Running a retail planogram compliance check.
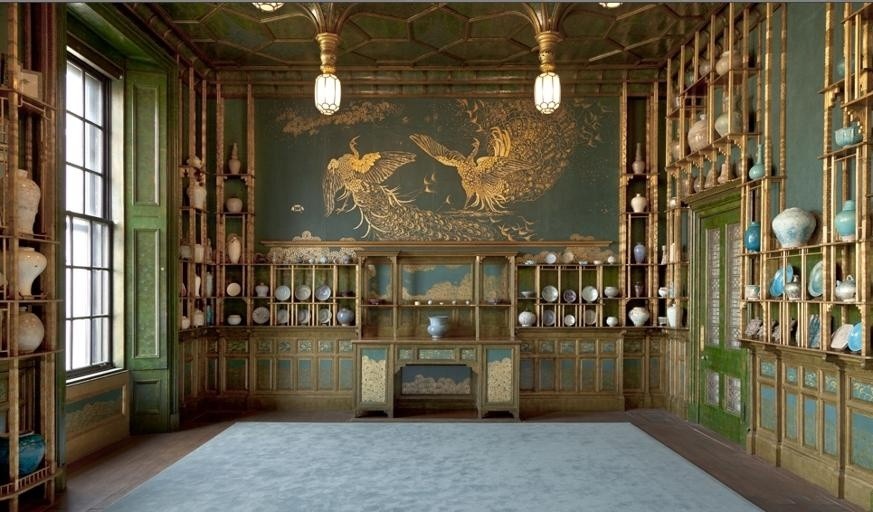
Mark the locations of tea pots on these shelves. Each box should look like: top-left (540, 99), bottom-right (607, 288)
top-left (835, 274), bottom-right (857, 302)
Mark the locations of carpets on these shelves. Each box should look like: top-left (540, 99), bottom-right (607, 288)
top-left (89, 421), bottom-right (763, 511)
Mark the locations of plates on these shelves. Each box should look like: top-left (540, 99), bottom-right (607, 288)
top-left (848, 321), bottom-right (862, 352)
top-left (277, 309), bottom-right (290, 324)
top-left (252, 306), bottom-right (270, 324)
top-left (584, 309), bottom-right (596, 325)
top-left (769, 264), bottom-right (794, 297)
top-left (315, 283), bottom-right (332, 301)
top-left (559, 250), bottom-right (574, 264)
top-left (542, 285), bottom-right (559, 303)
top-left (274, 285), bottom-right (290, 301)
top-left (808, 260), bottom-right (825, 298)
top-left (298, 309), bottom-right (312, 324)
top-left (582, 286), bottom-right (599, 302)
top-left (562, 288), bottom-right (577, 302)
top-left (830, 324), bottom-right (853, 351)
top-left (541, 309), bottom-right (555, 326)
top-left (318, 308), bottom-right (332, 323)
top-left (564, 314), bottom-right (576, 326)
top-left (227, 282), bottom-right (241, 296)
top-left (544, 253), bottom-right (557, 264)
top-left (295, 285), bottom-right (312, 300)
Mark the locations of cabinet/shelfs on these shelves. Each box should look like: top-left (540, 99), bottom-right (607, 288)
top-left (1, 1), bottom-right (63, 511)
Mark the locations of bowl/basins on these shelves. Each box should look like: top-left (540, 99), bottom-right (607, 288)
top-left (341, 290), bottom-right (353, 297)
top-left (521, 291), bottom-right (535, 298)
top-left (834, 124), bottom-right (864, 148)
top-left (368, 298), bottom-right (383, 304)
